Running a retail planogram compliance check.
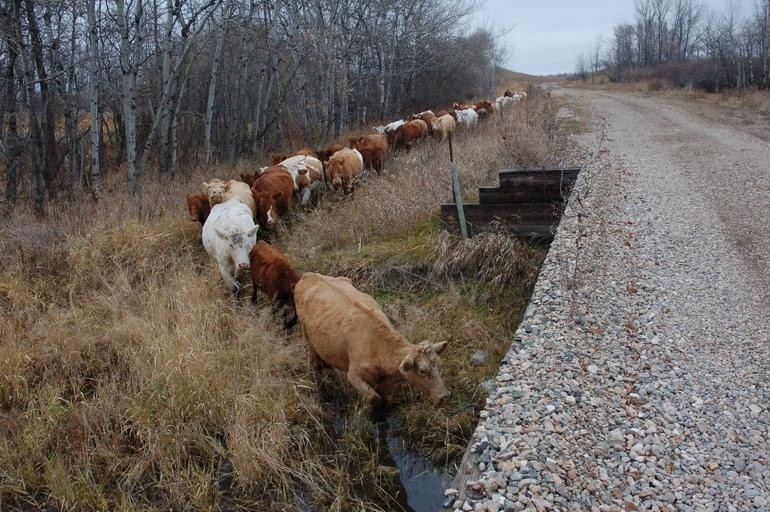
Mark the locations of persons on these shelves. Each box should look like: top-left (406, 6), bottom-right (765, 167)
top-left (504, 89), bottom-right (512, 96)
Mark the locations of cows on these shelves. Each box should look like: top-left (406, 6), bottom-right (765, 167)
top-left (348, 90), bottom-right (525, 179)
top-left (250, 239), bottom-right (303, 330)
top-left (186, 142), bottom-right (363, 225)
top-left (292, 269), bottom-right (451, 419)
top-left (201, 198), bottom-right (259, 303)
